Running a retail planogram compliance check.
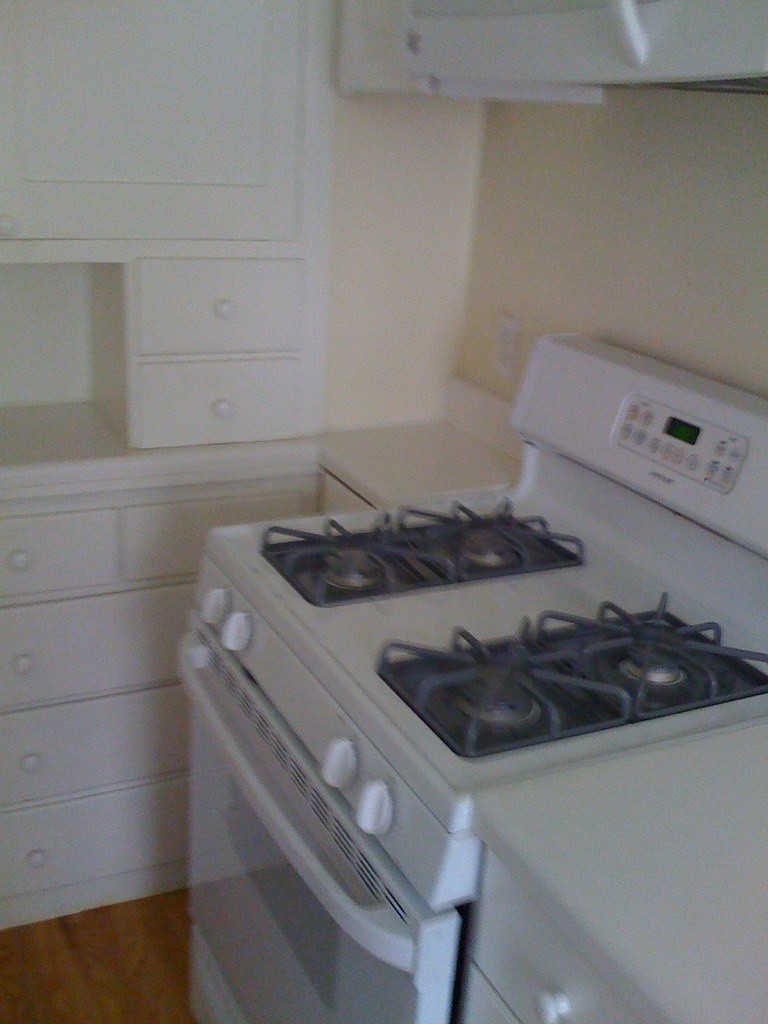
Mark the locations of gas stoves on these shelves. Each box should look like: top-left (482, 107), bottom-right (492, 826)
top-left (252, 328), bottom-right (768, 791)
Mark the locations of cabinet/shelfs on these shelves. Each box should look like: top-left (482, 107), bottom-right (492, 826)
top-left (318, 464), bottom-right (378, 521)
top-left (0, 0), bottom-right (332, 242)
top-left (461, 844), bottom-right (660, 1024)
top-left (133, 254), bottom-right (317, 450)
top-left (0, 473), bottom-right (319, 932)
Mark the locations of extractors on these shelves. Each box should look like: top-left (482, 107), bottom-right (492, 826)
top-left (396, 0), bottom-right (768, 95)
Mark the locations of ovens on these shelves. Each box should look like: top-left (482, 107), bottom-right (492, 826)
top-left (174, 608), bottom-right (467, 1024)
